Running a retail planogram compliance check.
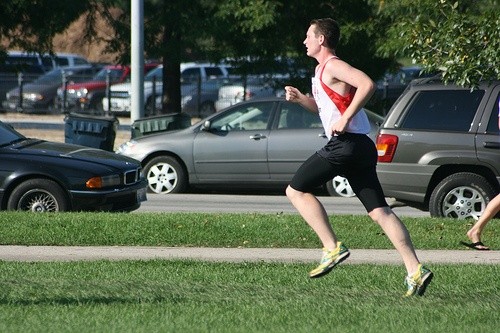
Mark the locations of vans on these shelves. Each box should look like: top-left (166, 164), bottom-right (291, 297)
top-left (0, 51), bottom-right (89, 116)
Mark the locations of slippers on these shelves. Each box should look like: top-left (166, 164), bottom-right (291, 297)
top-left (460, 241), bottom-right (489, 250)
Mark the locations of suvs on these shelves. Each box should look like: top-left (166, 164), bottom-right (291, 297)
top-left (57, 66), bottom-right (131, 113)
top-left (375, 71), bottom-right (500, 218)
top-left (101, 64), bottom-right (229, 116)
top-left (392, 67), bottom-right (443, 85)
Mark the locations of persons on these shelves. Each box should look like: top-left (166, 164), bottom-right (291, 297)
top-left (460, 193), bottom-right (500, 250)
top-left (285, 19), bottom-right (434, 298)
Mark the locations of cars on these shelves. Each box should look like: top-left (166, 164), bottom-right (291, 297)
top-left (2, 66), bottom-right (101, 114)
top-left (144, 77), bottom-right (256, 119)
top-left (116, 98), bottom-right (385, 198)
top-left (0, 121), bottom-right (149, 214)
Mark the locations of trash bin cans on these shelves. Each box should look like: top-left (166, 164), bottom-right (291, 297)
top-left (129, 112), bottom-right (192, 141)
top-left (64, 112), bottom-right (116, 151)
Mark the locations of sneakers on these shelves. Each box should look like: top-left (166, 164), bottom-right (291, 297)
top-left (310, 242), bottom-right (350, 278)
top-left (404, 264), bottom-right (433, 297)
